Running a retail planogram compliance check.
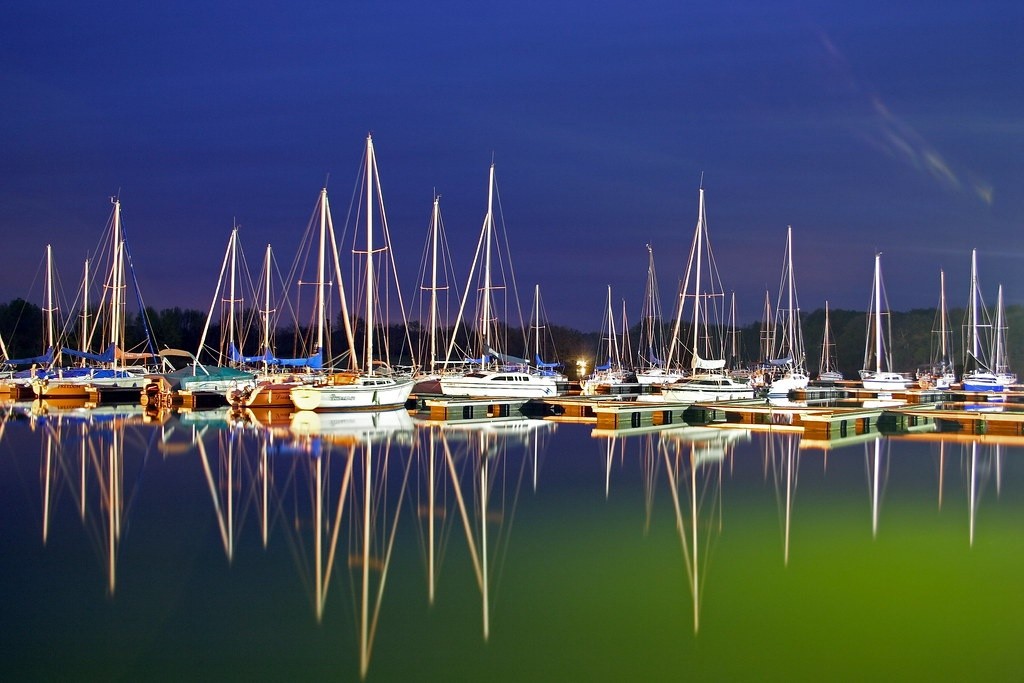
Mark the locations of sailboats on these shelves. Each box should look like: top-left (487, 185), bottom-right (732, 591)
top-left (1, 397), bottom-right (1024, 680)
top-left (1, 131), bottom-right (1024, 409)
